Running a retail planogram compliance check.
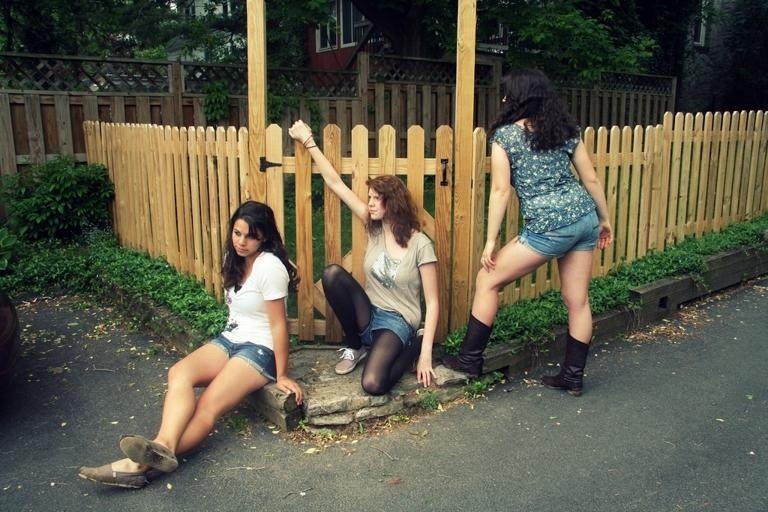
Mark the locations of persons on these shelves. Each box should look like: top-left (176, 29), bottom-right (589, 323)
top-left (77, 201), bottom-right (303, 490)
top-left (287, 119), bottom-right (440, 396)
top-left (440, 65), bottom-right (614, 398)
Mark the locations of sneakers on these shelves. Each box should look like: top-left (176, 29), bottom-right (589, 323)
top-left (416, 328), bottom-right (424, 335)
top-left (78, 434), bottom-right (179, 490)
top-left (335, 346), bottom-right (367, 375)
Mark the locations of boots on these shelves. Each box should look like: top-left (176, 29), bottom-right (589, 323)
top-left (442, 314), bottom-right (492, 377)
top-left (542, 335), bottom-right (589, 396)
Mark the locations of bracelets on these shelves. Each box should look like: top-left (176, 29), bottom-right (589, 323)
top-left (303, 135), bottom-right (317, 149)
